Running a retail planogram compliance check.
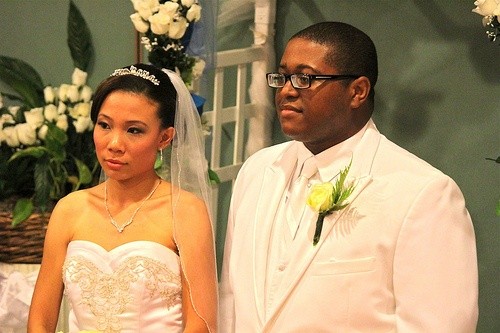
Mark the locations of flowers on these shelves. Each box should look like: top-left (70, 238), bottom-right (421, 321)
top-left (305, 152), bottom-right (358, 245)
top-left (127, 0), bottom-right (206, 91)
top-left (0, 0), bottom-right (109, 227)
top-left (472, 0), bottom-right (499, 41)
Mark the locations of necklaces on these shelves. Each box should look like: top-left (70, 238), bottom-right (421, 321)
top-left (104, 177), bottom-right (161, 233)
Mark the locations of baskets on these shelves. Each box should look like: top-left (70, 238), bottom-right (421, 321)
top-left (0, 201), bottom-right (56, 264)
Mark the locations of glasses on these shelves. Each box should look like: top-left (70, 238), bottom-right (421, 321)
top-left (265, 71), bottom-right (362, 90)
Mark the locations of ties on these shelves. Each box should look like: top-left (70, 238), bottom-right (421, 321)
top-left (286, 154), bottom-right (319, 240)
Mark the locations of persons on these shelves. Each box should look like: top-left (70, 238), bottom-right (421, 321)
top-left (216, 22), bottom-right (479, 333)
top-left (27, 62), bottom-right (223, 333)
